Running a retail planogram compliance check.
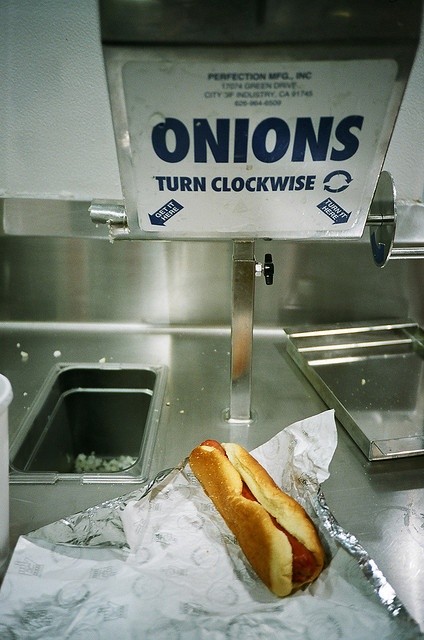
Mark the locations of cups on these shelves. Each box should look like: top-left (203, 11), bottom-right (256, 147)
top-left (1, 373), bottom-right (15, 563)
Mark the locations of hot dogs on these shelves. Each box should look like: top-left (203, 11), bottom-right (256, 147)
top-left (189, 438), bottom-right (328, 601)
